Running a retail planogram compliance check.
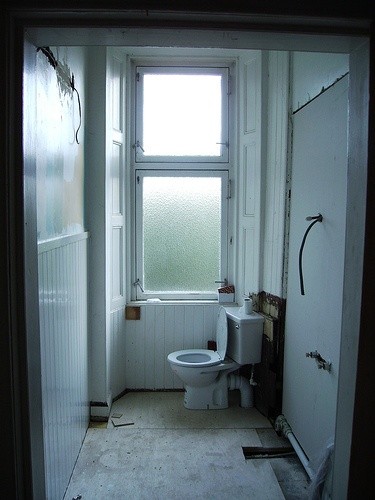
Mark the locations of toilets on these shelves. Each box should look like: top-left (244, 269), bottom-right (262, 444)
top-left (166, 306), bottom-right (265, 409)
